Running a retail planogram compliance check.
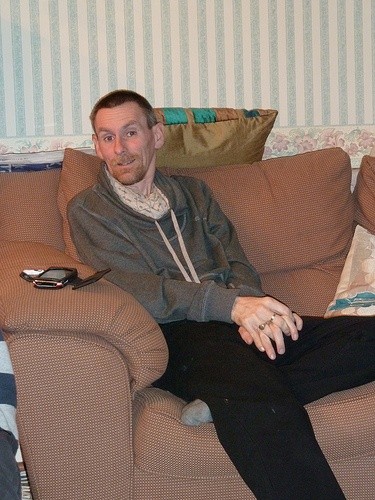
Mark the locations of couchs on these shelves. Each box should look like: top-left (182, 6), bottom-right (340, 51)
top-left (0, 148), bottom-right (375, 500)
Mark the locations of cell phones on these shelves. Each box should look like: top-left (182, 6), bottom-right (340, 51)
top-left (33, 265), bottom-right (78, 290)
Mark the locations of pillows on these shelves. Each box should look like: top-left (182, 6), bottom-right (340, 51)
top-left (152, 107), bottom-right (279, 168)
top-left (323, 224), bottom-right (375, 317)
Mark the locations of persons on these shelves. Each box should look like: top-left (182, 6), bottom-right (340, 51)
top-left (66, 90), bottom-right (375, 500)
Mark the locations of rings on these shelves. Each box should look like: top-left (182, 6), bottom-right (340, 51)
top-left (259, 320), bottom-right (271, 330)
top-left (270, 312), bottom-right (278, 322)
